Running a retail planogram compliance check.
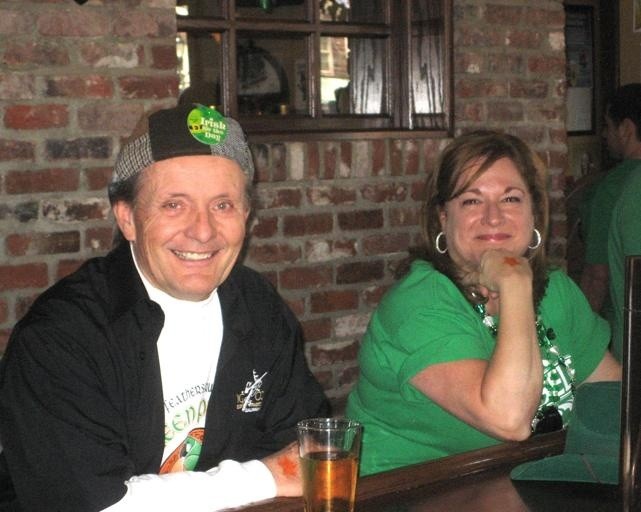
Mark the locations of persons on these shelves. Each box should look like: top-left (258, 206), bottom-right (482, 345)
top-left (333, 130), bottom-right (625, 480)
top-left (575, 82), bottom-right (640, 366)
top-left (605, 164), bottom-right (641, 366)
top-left (0, 99), bottom-right (338, 512)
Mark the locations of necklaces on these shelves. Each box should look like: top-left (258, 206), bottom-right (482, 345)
top-left (468, 289), bottom-right (553, 356)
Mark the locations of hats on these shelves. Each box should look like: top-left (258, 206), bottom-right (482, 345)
top-left (112, 103), bottom-right (255, 185)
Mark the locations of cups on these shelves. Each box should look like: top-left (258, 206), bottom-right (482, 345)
top-left (294, 417), bottom-right (363, 512)
